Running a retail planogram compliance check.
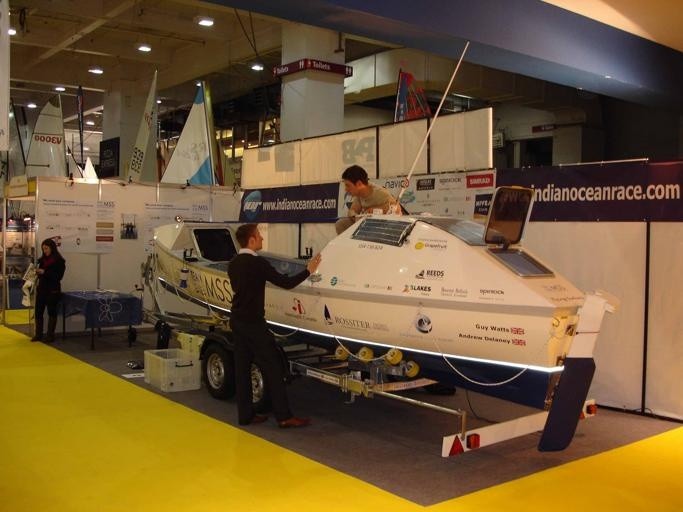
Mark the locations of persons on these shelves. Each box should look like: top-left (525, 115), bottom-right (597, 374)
top-left (333, 164), bottom-right (403, 235)
top-left (225, 221), bottom-right (321, 429)
top-left (29, 238), bottom-right (67, 342)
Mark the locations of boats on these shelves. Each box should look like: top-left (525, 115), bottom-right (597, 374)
top-left (147, 185), bottom-right (621, 457)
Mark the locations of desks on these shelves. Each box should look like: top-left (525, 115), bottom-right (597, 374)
top-left (61, 290), bottom-right (142, 350)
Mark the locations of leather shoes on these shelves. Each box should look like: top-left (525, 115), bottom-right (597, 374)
top-left (244, 412), bottom-right (313, 428)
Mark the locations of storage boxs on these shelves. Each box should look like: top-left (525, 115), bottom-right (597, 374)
top-left (142, 348), bottom-right (202, 394)
